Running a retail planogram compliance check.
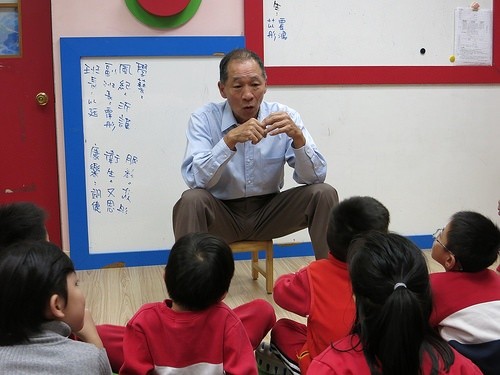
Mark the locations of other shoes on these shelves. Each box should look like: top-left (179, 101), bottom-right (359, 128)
top-left (254, 342), bottom-right (294, 375)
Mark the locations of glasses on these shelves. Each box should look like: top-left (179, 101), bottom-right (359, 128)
top-left (433, 228), bottom-right (455, 257)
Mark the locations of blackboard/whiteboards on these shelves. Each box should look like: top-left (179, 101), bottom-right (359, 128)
top-left (242, 0), bottom-right (500, 85)
top-left (58, 36), bottom-right (500, 272)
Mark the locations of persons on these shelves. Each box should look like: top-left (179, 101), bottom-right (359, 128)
top-left (95, 233), bottom-right (276, 375)
top-left (305, 232), bottom-right (485, 375)
top-left (0, 242), bottom-right (113, 375)
top-left (0, 201), bottom-right (49, 242)
top-left (171, 49), bottom-right (339, 261)
top-left (269, 196), bottom-right (390, 375)
top-left (428, 211), bottom-right (500, 375)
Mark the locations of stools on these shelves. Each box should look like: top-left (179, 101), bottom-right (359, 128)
top-left (229, 240), bottom-right (274, 293)
